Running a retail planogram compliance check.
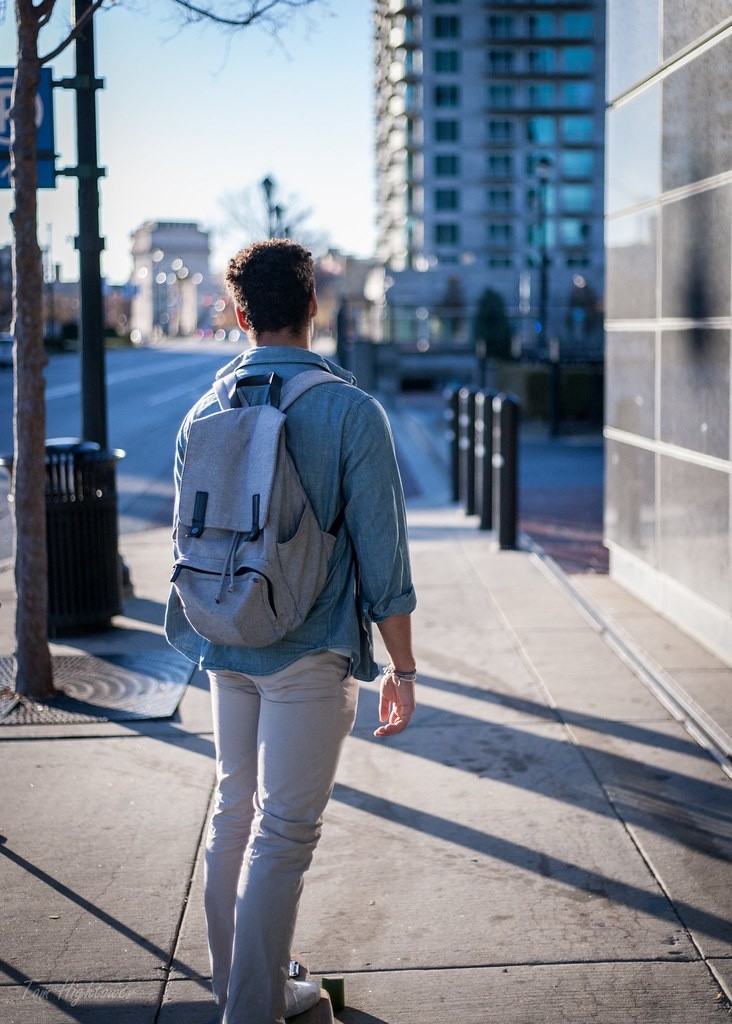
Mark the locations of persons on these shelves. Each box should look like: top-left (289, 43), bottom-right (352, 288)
top-left (156, 240), bottom-right (418, 1024)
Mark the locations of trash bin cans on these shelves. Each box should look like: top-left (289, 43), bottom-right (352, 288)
top-left (0, 438), bottom-right (127, 639)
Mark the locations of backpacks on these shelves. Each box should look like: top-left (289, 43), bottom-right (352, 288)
top-left (165, 371), bottom-right (352, 648)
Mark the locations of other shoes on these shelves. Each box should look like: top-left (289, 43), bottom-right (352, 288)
top-left (285, 981), bottom-right (320, 1018)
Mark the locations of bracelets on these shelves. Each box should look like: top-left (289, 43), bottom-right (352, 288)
top-left (387, 664), bottom-right (417, 685)
